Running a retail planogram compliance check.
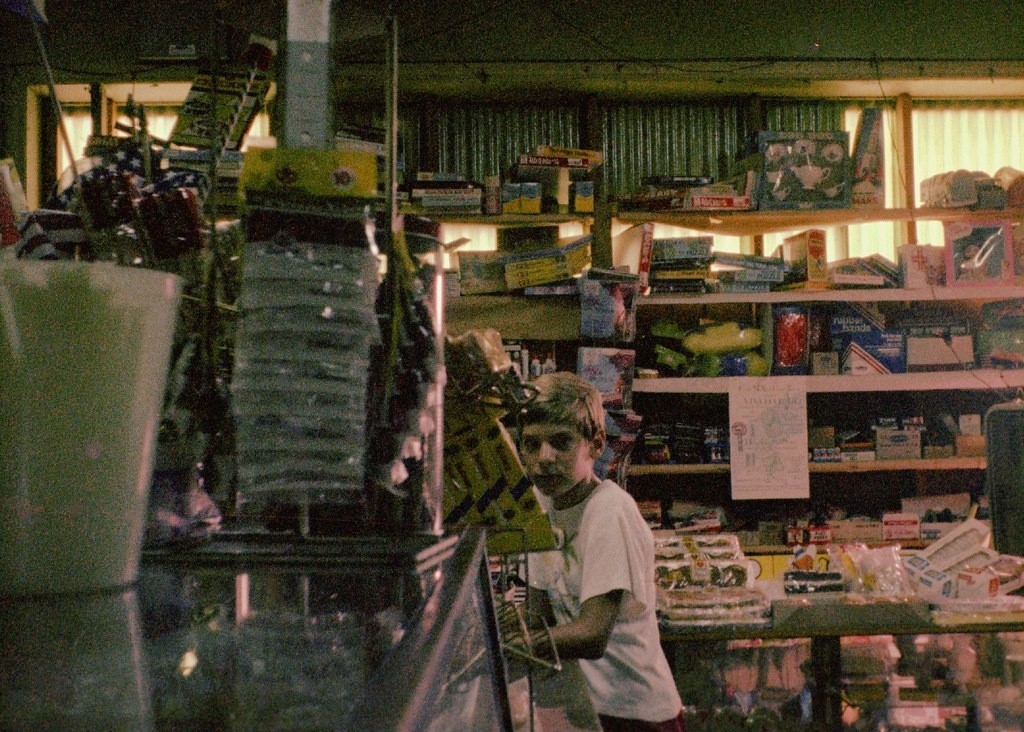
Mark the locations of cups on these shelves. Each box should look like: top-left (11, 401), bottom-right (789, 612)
top-left (0, 589), bottom-right (155, 732)
top-left (0, 260), bottom-right (186, 600)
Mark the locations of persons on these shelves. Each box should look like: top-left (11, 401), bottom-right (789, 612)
top-left (456, 370), bottom-right (686, 732)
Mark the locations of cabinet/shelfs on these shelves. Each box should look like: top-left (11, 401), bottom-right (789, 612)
top-left (431, 206), bottom-right (1024, 477)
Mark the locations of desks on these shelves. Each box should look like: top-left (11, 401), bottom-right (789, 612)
top-left (657, 607), bottom-right (1024, 732)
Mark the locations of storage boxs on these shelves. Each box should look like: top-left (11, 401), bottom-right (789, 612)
top-left (333, 106), bottom-right (1024, 603)
